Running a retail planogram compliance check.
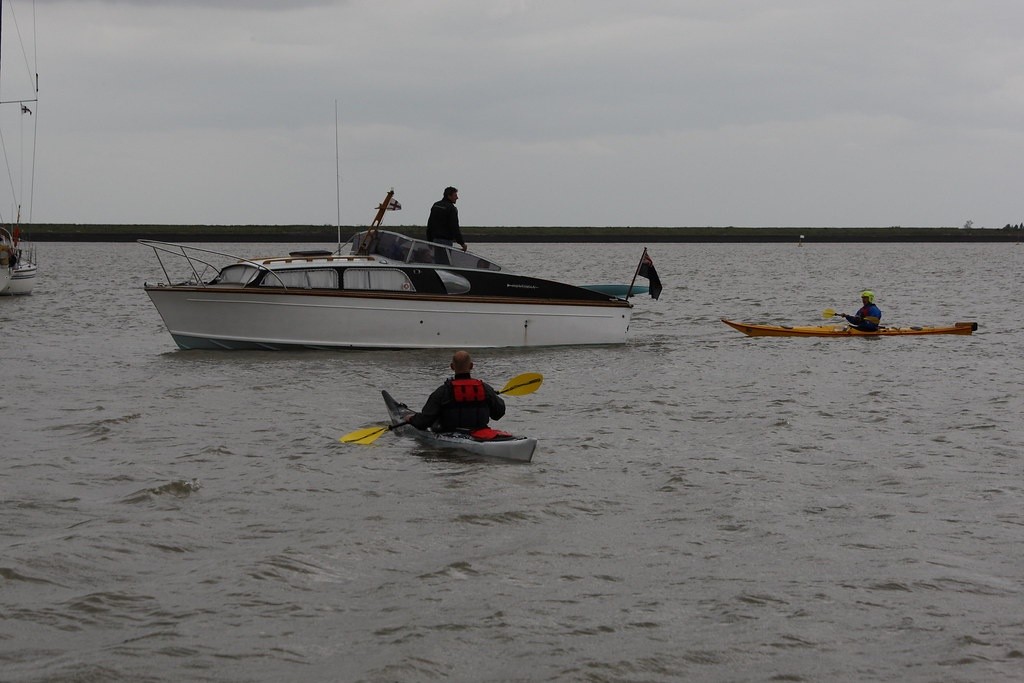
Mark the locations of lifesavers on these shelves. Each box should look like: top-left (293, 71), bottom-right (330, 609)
top-left (0, 246), bottom-right (11, 265)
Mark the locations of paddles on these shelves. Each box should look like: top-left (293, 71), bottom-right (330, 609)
top-left (823, 308), bottom-right (880, 324)
top-left (340, 372), bottom-right (544, 446)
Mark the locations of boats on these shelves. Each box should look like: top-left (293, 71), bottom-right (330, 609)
top-left (718, 314), bottom-right (979, 339)
top-left (381, 389), bottom-right (540, 463)
top-left (132, 98), bottom-right (638, 351)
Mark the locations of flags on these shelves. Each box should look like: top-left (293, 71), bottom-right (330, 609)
top-left (387, 199), bottom-right (401, 211)
top-left (638, 252), bottom-right (663, 301)
top-left (22, 106), bottom-right (32, 115)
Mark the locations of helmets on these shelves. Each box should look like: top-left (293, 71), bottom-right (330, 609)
top-left (861, 290), bottom-right (873, 303)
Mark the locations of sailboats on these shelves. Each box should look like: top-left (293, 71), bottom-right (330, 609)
top-left (0, 2), bottom-right (50, 297)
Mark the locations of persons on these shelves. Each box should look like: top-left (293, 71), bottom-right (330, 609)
top-left (404, 350), bottom-right (506, 435)
top-left (841, 291), bottom-right (882, 331)
top-left (426, 186), bottom-right (467, 265)
